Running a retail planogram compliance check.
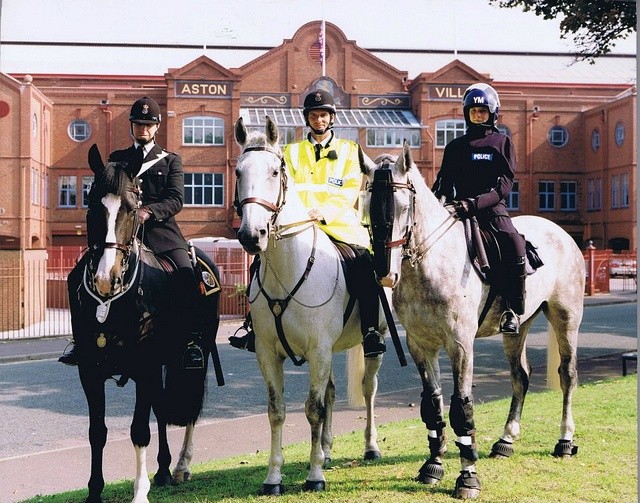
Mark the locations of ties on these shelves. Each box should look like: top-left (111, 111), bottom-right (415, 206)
top-left (315, 144), bottom-right (323, 162)
top-left (130, 145), bottom-right (144, 180)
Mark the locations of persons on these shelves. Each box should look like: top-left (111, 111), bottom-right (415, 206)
top-left (227, 89), bottom-right (386, 358)
top-left (56, 96), bottom-right (206, 369)
top-left (431, 83), bottom-right (527, 336)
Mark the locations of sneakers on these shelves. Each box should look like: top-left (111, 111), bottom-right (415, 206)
top-left (227, 329), bottom-right (256, 353)
top-left (58, 345), bottom-right (78, 363)
top-left (184, 345), bottom-right (208, 367)
top-left (502, 314), bottom-right (520, 332)
top-left (363, 335), bottom-right (386, 358)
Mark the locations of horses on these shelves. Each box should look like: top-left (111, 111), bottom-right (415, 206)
top-left (67, 142), bottom-right (223, 503)
top-left (233, 115), bottom-right (397, 498)
top-left (355, 138), bottom-right (587, 501)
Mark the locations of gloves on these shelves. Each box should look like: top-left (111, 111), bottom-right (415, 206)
top-left (452, 200), bottom-right (474, 215)
top-left (308, 208), bottom-right (324, 223)
top-left (137, 206), bottom-right (153, 224)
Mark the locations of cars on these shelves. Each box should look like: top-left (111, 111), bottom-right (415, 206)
top-left (610, 258), bottom-right (637, 279)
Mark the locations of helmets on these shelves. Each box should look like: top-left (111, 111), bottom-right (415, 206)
top-left (129, 96), bottom-right (162, 125)
top-left (302, 91), bottom-right (336, 117)
top-left (462, 82), bottom-right (501, 127)
